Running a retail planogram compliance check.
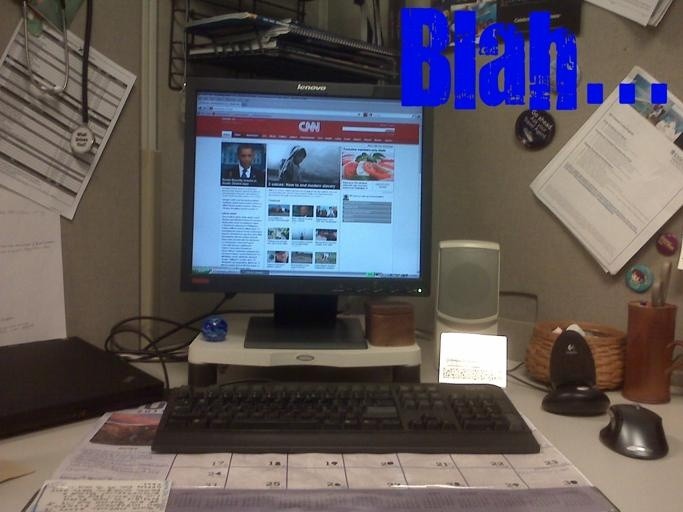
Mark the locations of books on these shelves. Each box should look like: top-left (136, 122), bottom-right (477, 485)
top-left (183, 12), bottom-right (396, 78)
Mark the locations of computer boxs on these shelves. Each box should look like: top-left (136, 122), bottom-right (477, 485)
top-left (188, 311), bottom-right (422, 390)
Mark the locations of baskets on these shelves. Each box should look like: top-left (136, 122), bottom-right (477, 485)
top-left (528, 321), bottom-right (626, 391)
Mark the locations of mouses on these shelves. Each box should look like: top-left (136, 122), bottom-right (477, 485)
top-left (599, 404), bottom-right (669, 460)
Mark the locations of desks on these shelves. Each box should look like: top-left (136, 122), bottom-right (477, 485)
top-left (0, 350), bottom-right (683, 512)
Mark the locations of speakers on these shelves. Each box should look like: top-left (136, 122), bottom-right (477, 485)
top-left (434, 239), bottom-right (501, 368)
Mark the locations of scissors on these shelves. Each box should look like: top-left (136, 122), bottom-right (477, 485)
top-left (651, 261), bottom-right (673, 308)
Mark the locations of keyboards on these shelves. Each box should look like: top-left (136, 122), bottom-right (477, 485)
top-left (151, 383), bottom-right (542, 454)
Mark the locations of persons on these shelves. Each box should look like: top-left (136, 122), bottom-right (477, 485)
top-left (278, 144), bottom-right (308, 184)
top-left (277, 251), bottom-right (287, 264)
top-left (317, 230), bottom-right (327, 239)
top-left (296, 207), bottom-right (311, 215)
top-left (326, 230), bottom-right (337, 241)
top-left (224, 144), bottom-right (264, 187)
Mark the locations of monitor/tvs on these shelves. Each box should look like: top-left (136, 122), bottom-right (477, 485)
top-left (180, 75), bottom-right (434, 351)
